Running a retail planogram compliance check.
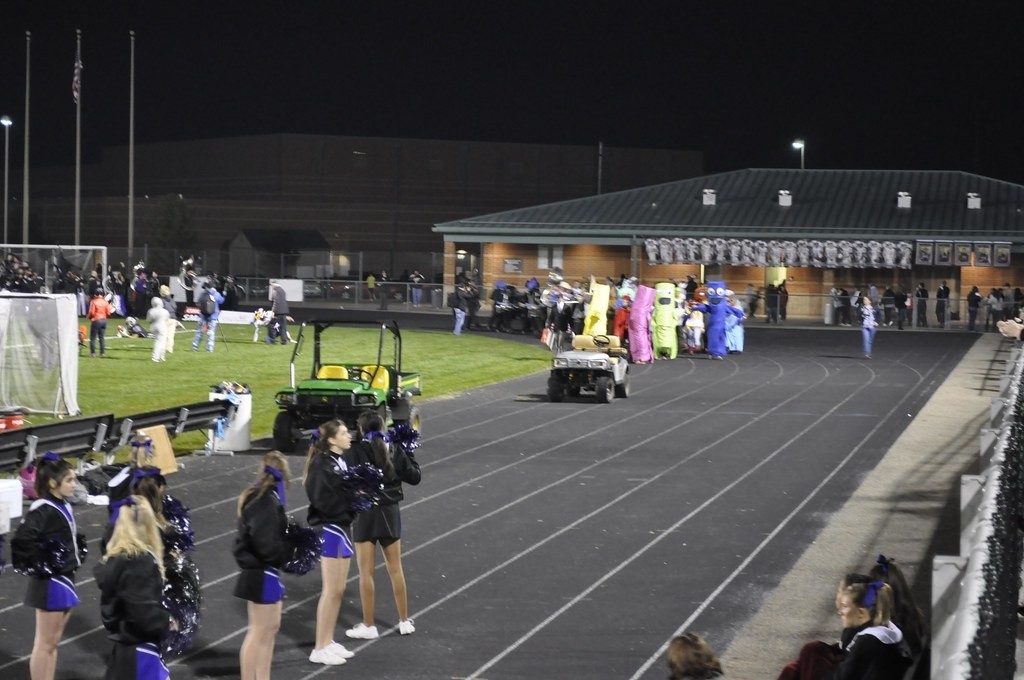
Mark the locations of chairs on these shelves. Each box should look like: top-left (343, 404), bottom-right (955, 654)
top-left (597, 335), bottom-right (621, 364)
top-left (574, 335), bottom-right (595, 350)
top-left (316, 365), bottom-right (349, 379)
top-left (361, 366), bottom-right (389, 393)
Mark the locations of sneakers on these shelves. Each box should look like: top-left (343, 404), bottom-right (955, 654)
top-left (309, 648), bottom-right (347, 666)
top-left (326, 639), bottom-right (355, 658)
top-left (398, 617), bottom-right (416, 635)
top-left (345, 622), bottom-right (379, 639)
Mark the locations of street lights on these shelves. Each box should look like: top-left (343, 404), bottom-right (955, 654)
top-left (0, 115), bottom-right (12, 246)
top-left (791, 138), bottom-right (805, 170)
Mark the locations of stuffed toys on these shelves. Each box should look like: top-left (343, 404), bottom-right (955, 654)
top-left (584, 280), bottom-right (746, 365)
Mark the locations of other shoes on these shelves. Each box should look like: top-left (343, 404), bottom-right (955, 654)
top-left (151, 356), bottom-right (166, 363)
top-left (289, 338), bottom-right (298, 343)
top-left (888, 320), bottom-right (893, 326)
top-left (89, 353), bottom-right (95, 358)
top-left (100, 353), bottom-right (105, 357)
top-left (898, 327), bottom-right (904, 330)
top-left (839, 323), bottom-right (845, 326)
top-left (883, 323), bottom-right (887, 326)
top-left (845, 323), bottom-right (851, 327)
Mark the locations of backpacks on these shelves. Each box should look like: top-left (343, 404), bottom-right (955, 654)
top-left (447, 290), bottom-right (459, 308)
top-left (201, 290), bottom-right (215, 316)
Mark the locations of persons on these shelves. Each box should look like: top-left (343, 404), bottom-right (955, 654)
top-left (0, 254), bottom-right (225, 361)
top-left (748, 280), bottom-right (788, 324)
top-left (94, 434), bottom-right (177, 680)
top-left (921, 248), bottom-right (1008, 263)
top-left (667, 633), bottom-right (723, 680)
top-left (607, 273), bottom-right (627, 286)
top-left (831, 282), bottom-right (928, 330)
top-left (686, 275), bottom-right (697, 299)
top-left (267, 283), bottom-right (297, 344)
top-left (366, 270), bottom-right (389, 310)
top-left (345, 410), bottom-right (422, 639)
top-left (967, 283), bottom-right (1024, 332)
top-left (231, 451), bottom-right (292, 680)
top-left (303, 421), bottom-right (356, 666)
top-left (936, 281), bottom-right (950, 328)
top-left (409, 270), bottom-right (424, 308)
top-left (859, 297), bottom-right (881, 359)
top-left (778, 554), bottom-right (925, 680)
top-left (21, 452), bottom-right (80, 680)
top-left (454, 278), bottom-right (585, 354)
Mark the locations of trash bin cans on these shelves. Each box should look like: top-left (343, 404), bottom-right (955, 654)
top-left (208, 392), bottom-right (252, 451)
top-left (824, 303), bottom-right (835, 325)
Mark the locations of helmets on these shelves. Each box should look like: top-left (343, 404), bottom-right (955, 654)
top-left (126, 317), bottom-right (135, 327)
top-left (160, 284), bottom-right (170, 298)
